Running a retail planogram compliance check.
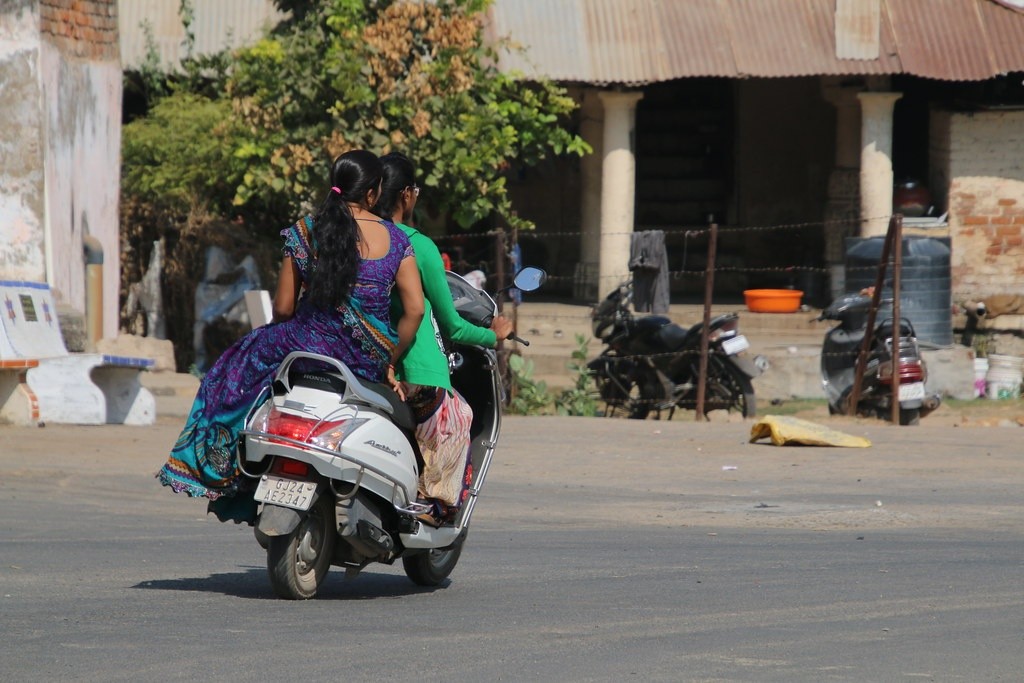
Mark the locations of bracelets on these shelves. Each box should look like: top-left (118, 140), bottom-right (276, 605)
top-left (388, 363), bottom-right (397, 371)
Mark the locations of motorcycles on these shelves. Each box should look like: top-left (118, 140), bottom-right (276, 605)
top-left (587, 281), bottom-right (771, 421)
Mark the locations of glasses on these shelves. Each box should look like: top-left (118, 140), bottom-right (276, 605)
top-left (400, 185), bottom-right (421, 197)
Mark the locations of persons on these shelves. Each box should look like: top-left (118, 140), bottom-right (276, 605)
top-left (155, 150), bottom-right (514, 528)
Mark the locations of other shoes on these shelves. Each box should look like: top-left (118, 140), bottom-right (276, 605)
top-left (208, 491), bottom-right (260, 526)
top-left (425, 496), bottom-right (447, 516)
top-left (415, 496), bottom-right (447, 528)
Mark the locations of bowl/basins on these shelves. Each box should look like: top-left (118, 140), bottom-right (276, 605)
top-left (742, 289), bottom-right (803, 313)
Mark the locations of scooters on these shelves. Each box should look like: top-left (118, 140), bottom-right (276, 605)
top-left (808, 288), bottom-right (942, 427)
top-left (237, 268), bottom-right (549, 600)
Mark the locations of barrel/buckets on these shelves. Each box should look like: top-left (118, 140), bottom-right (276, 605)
top-left (986, 354), bottom-right (1024, 400)
top-left (974, 358), bottom-right (988, 398)
